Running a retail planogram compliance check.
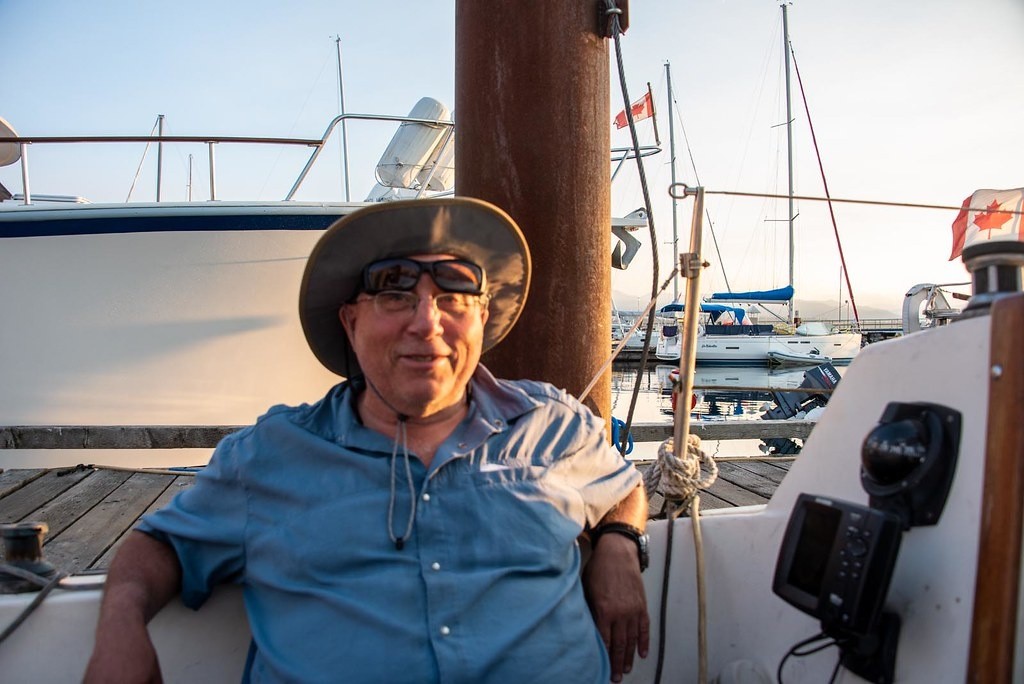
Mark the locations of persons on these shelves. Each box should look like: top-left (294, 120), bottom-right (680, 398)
top-left (79, 196), bottom-right (654, 684)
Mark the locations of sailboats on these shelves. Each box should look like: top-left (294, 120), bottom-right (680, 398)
top-left (654, 0), bottom-right (864, 367)
top-left (654, 362), bottom-right (852, 422)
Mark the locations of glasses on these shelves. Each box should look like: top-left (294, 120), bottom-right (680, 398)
top-left (347, 256), bottom-right (487, 315)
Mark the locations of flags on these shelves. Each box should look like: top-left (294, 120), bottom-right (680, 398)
top-left (947, 188), bottom-right (1024, 262)
top-left (613, 89), bottom-right (656, 129)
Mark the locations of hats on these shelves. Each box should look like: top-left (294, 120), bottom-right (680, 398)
top-left (298, 196), bottom-right (531, 378)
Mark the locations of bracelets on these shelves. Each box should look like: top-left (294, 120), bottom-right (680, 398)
top-left (592, 521), bottom-right (650, 573)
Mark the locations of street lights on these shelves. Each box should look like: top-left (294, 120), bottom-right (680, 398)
top-left (845, 300), bottom-right (850, 332)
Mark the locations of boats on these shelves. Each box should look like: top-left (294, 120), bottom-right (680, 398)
top-left (1, 30), bottom-right (665, 470)
top-left (610, 294), bottom-right (675, 352)
top-left (767, 349), bottom-right (834, 365)
